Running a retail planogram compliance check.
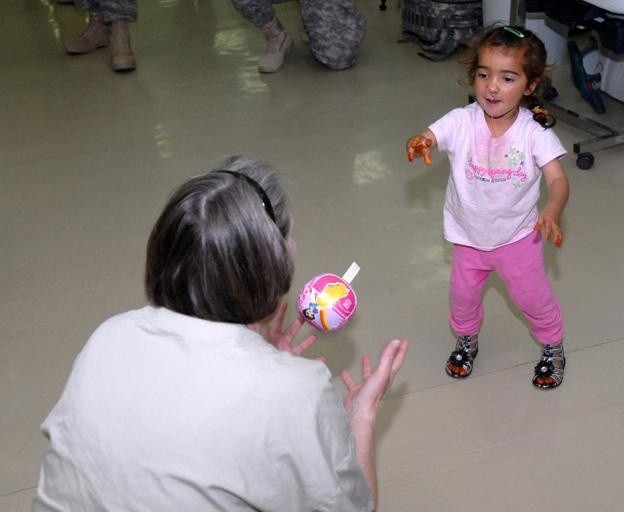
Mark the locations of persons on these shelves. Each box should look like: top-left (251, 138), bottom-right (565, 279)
top-left (65, 0), bottom-right (138, 72)
top-left (230, 0), bottom-right (367, 74)
top-left (30, 153), bottom-right (408, 511)
top-left (406, 23), bottom-right (569, 390)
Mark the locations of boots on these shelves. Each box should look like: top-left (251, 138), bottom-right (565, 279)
top-left (259, 17), bottom-right (295, 74)
top-left (66, 8), bottom-right (110, 54)
top-left (109, 19), bottom-right (136, 74)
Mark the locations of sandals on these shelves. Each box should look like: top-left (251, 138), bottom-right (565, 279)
top-left (446, 336), bottom-right (479, 378)
top-left (532, 343), bottom-right (565, 389)
